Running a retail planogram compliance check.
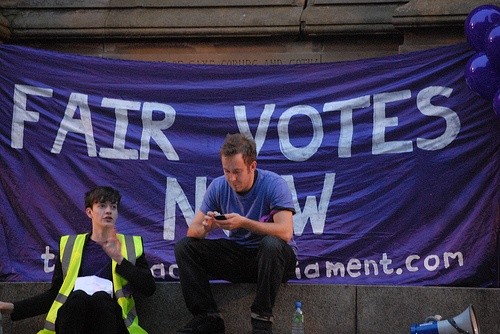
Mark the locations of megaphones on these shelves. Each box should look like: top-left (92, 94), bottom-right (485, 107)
top-left (412, 302), bottom-right (479, 334)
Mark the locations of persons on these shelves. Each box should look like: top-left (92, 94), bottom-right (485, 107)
top-left (0, 186), bottom-right (157, 334)
top-left (174, 134), bottom-right (299, 334)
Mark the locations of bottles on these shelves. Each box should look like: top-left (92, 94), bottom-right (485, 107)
top-left (290, 301), bottom-right (305, 334)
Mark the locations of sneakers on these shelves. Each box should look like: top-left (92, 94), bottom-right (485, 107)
top-left (250, 318), bottom-right (273, 333)
top-left (177, 316), bottom-right (225, 334)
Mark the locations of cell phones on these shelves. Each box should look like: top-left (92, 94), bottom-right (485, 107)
top-left (215, 215), bottom-right (226, 220)
